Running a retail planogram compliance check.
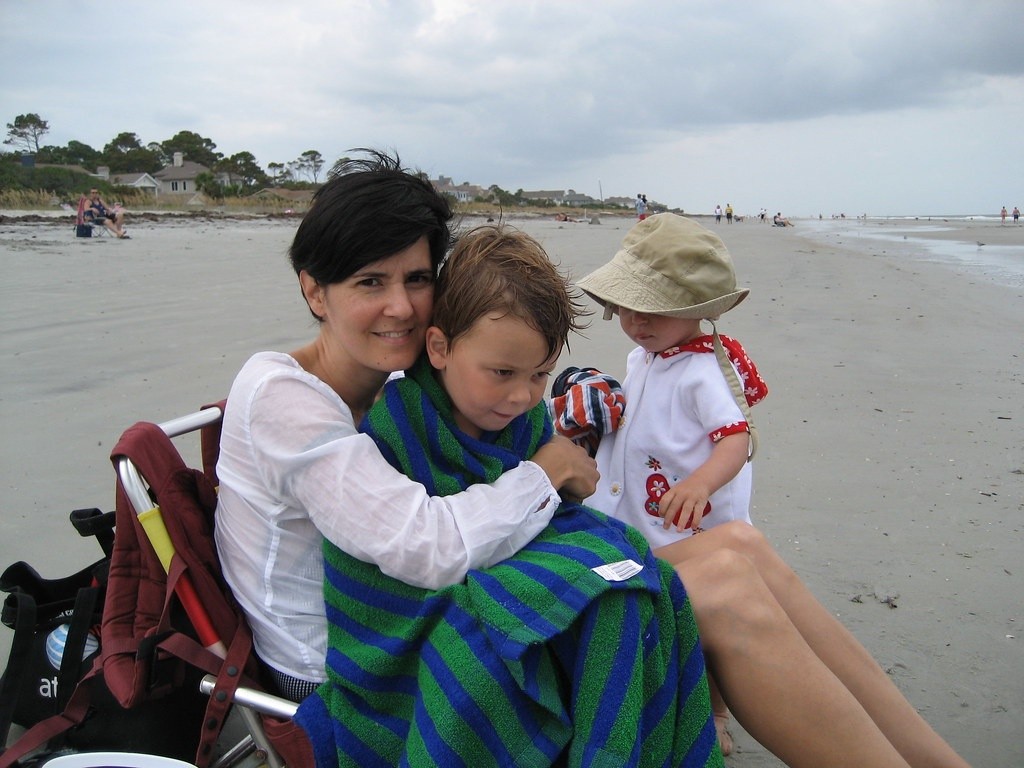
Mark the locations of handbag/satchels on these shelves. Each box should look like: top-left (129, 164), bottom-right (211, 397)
top-left (0, 507), bottom-right (231, 760)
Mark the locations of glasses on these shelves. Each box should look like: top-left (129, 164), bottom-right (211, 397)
top-left (91, 192), bottom-right (98, 194)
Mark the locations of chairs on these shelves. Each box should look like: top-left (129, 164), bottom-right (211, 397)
top-left (115, 399), bottom-right (324, 768)
top-left (79, 196), bottom-right (113, 238)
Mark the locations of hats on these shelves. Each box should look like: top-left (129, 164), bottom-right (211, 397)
top-left (575, 212), bottom-right (751, 321)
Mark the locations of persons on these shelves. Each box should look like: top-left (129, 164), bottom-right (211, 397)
top-left (1011, 207), bottom-right (1020, 221)
top-left (575, 212), bottom-right (768, 753)
top-left (758, 208), bottom-right (767, 224)
top-left (214, 150), bottom-right (970, 768)
top-left (725, 204), bottom-right (733, 224)
top-left (293, 226), bottom-right (721, 768)
top-left (773, 212), bottom-right (794, 227)
top-left (636, 194), bottom-right (648, 220)
top-left (84, 188), bottom-right (129, 239)
top-left (1000, 206), bottom-right (1007, 223)
top-left (715, 205), bottom-right (722, 224)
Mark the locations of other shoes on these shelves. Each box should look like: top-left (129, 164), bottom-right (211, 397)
top-left (120, 231), bottom-right (130, 239)
